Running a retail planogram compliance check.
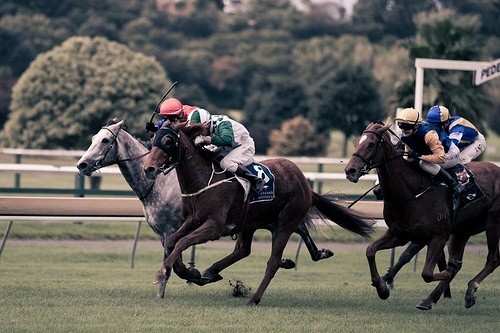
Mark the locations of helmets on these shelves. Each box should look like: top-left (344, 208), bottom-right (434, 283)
top-left (394, 107), bottom-right (423, 125)
top-left (158, 98), bottom-right (184, 115)
top-left (185, 108), bottom-right (212, 127)
top-left (427, 105), bottom-right (451, 123)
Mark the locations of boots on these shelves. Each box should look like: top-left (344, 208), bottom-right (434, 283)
top-left (435, 167), bottom-right (465, 193)
top-left (234, 165), bottom-right (265, 195)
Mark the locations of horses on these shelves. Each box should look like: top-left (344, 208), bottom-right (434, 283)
top-left (345, 122), bottom-right (500, 311)
top-left (75, 117), bottom-right (379, 308)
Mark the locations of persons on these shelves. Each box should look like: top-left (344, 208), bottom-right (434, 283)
top-left (146, 98), bottom-right (265, 197)
top-left (394, 105), bottom-right (487, 211)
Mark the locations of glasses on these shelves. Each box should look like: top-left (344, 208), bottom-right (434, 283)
top-left (398, 122), bottom-right (415, 130)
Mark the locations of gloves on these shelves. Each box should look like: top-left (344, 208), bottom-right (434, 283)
top-left (194, 135), bottom-right (204, 146)
top-left (408, 150), bottom-right (423, 161)
top-left (146, 122), bottom-right (159, 133)
